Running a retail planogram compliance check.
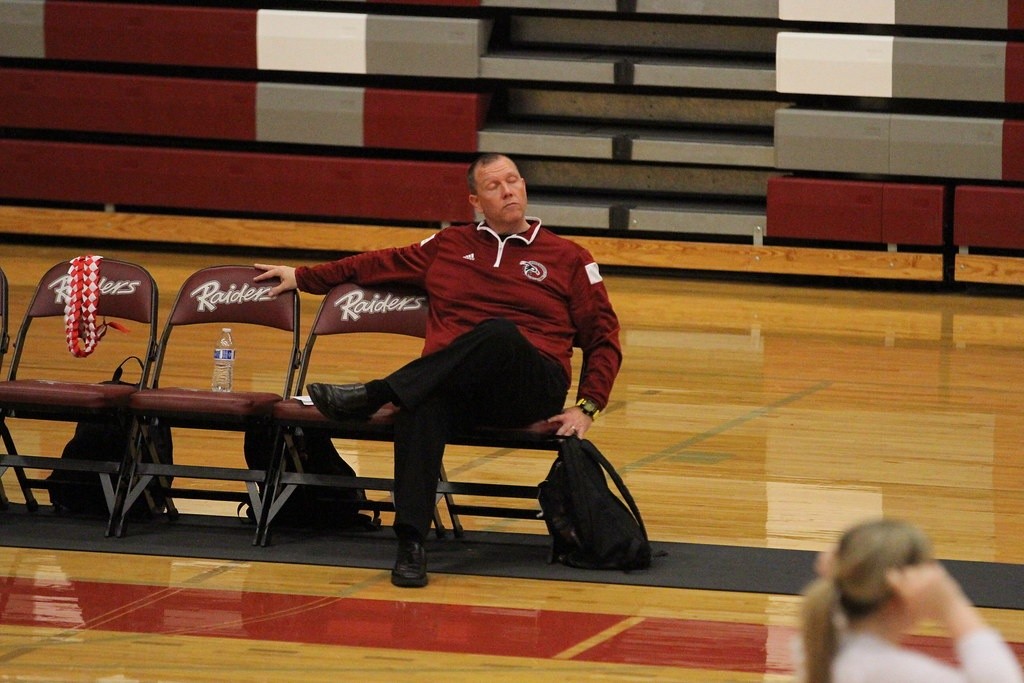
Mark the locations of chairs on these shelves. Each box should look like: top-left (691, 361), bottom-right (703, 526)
top-left (0, 254), bottom-right (589, 565)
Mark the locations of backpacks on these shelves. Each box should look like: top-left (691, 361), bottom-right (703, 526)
top-left (46, 355), bottom-right (173, 519)
top-left (539, 434), bottom-right (652, 571)
top-left (237, 430), bottom-right (368, 538)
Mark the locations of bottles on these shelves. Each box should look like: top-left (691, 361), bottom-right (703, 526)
top-left (211, 327), bottom-right (236, 392)
top-left (752, 224), bottom-right (763, 260)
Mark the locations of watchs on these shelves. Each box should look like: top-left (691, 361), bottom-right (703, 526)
top-left (576, 398), bottom-right (600, 422)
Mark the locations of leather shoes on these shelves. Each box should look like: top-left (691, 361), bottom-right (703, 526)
top-left (306, 382), bottom-right (371, 425)
top-left (391, 540), bottom-right (429, 588)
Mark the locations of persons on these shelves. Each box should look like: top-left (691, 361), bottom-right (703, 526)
top-left (253, 153), bottom-right (625, 589)
top-left (793, 518), bottom-right (1024, 682)
